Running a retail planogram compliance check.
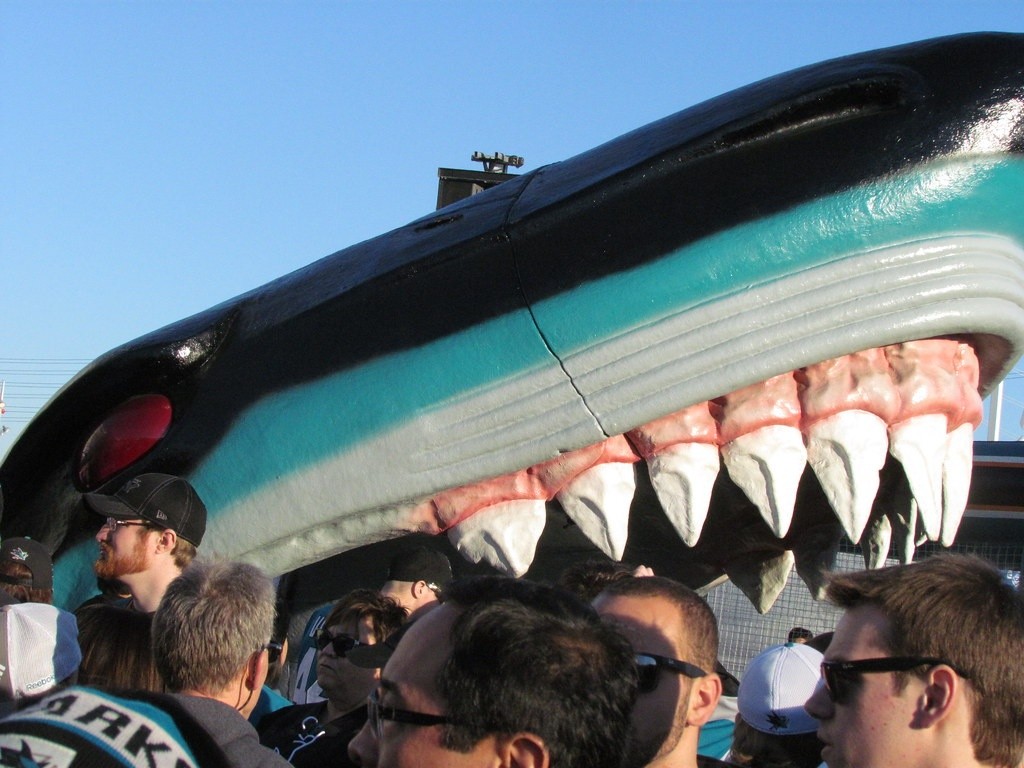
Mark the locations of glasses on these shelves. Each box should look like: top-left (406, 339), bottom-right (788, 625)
top-left (106, 517), bottom-right (160, 531)
top-left (633, 651), bottom-right (708, 692)
top-left (314, 629), bottom-right (373, 658)
top-left (255, 635), bottom-right (283, 663)
top-left (367, 695), bottom-right (513, 740)
top-left (819, 656), bottom-right (972, 705)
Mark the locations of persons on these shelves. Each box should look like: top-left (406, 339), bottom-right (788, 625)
top-left (0, 471), bottom-right (455, 768)
top-left (592, 575), bottom-right (722, 768)
top-left (353, 574), bottom-right (638, 768)
top-left (805, 555), bottom-right (1024, 768)
top-left (733, 626), bottom-right (834, 768)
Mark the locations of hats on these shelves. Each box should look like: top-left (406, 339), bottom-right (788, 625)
top-left (82, 473), bottom-right (207, 548)
top-left (0, 685), bottom-right (227, 768)
top-left (0, 537), bottom-right (53, 590)
top-left (738, 642), bottom-right (827, 735)
top-left (0, 601), bottom-right (82, 699)
top-left (345, 620), bottom-right (419, 668)
top-left (386, 545), bottom-right (452, 603)
